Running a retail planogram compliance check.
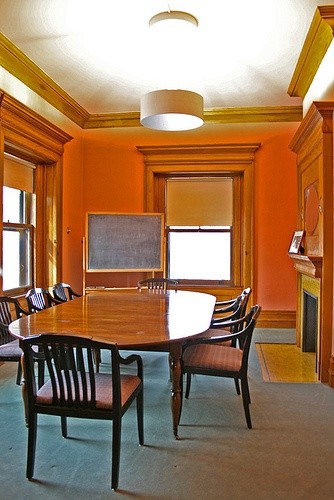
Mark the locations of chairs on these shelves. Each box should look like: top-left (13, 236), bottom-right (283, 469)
top-left (0, 283), bottom-right (92, 386)
top-left (138, 278), bottom-right (178, 289)
top-left (177, 304), bottom-right (261, 429)
top-left (211, 288), bottom-right (252, 350)
top-left (19, 334), bottom-right (144, 491)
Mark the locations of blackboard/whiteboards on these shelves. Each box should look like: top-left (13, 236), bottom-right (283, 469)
top-left (85, 211), bottom-right (165, 273)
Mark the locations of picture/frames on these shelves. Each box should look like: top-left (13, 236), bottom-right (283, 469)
top-left (288, 231), bottom-right (304, 254)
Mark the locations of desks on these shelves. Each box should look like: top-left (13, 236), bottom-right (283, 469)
top-left (8, 289), bottom-right (216, 439)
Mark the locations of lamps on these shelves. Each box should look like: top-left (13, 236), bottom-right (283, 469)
top-left (140, 11), bottom-right (204, 131)
